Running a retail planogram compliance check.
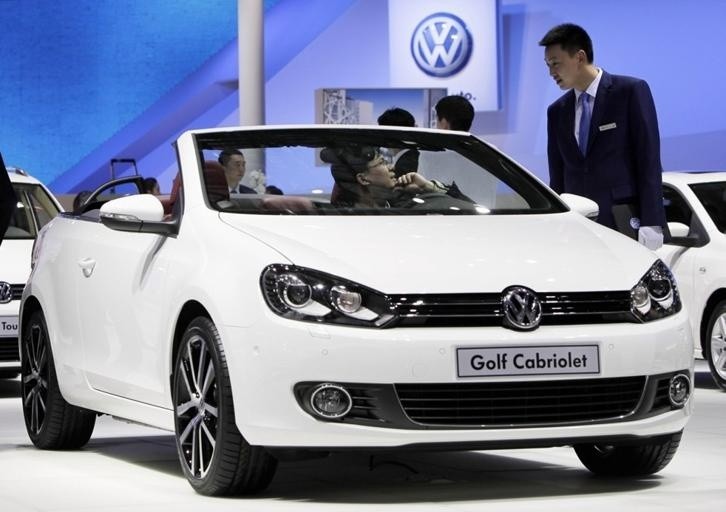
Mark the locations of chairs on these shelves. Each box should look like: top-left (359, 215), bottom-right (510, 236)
top-left (328, 184), bottom-right (357, 210)
top-left (169, 162), bottom-right (231, 210)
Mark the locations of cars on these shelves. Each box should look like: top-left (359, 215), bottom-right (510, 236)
top-left (654, 168), bottom-right (726, 392)
top-left (17, 123), bottom-right (696, 495)
top-left (0, 167), bottom-right (68, 379)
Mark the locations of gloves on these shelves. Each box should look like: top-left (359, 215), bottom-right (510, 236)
top-left (637, 223), bottom-right (665, 252)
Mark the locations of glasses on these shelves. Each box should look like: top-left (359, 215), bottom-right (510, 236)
top-left (361, 156), bottom-right (390, 175)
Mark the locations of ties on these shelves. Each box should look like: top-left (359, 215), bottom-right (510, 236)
top-left (578, 92), bottom-right (594, 159)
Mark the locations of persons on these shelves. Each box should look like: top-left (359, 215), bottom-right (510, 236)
top-left (143, 177), bottom-right (161, 195)
top-left (218, 151), bottom-right (257, 195)
top-left (320, 144), bottom-right (477, 208)
top-left (539, 21), bottom-right (672, 253)
top-left (266, 185), bottom-right (283, 195)
top-left (377, 108), bottom-right (420, 178)
top-left (419, 95), bottom-right (498, 209)
